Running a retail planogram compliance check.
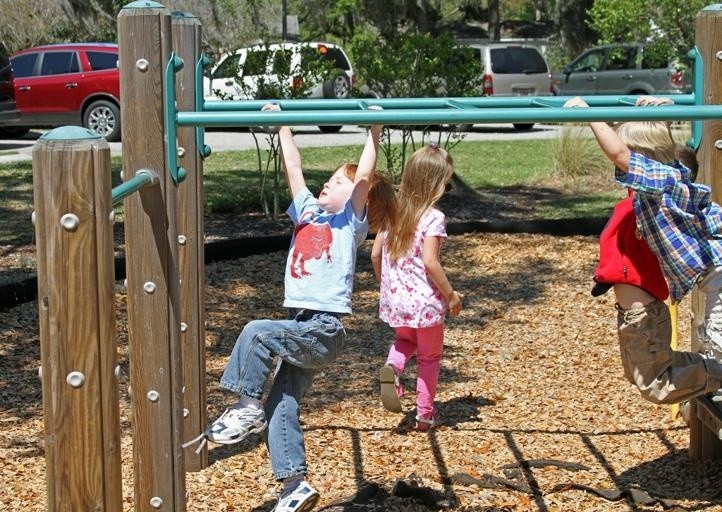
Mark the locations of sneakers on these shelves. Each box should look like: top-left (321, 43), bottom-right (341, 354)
top-left (205, 407), bottom-right (267, 446)
top-left (267, 481), bottom-right (317, 512)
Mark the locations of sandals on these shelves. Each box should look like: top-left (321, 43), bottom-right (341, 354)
top-left (380, 365), bottom-right (401, 415)
top-left (414, 413), bottom-right (443, 431)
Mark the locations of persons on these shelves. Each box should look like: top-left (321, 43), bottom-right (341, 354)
top-left (591, 93), bottom-right (722, 407)
top-left (181, 102), bottom-right (402, 512)
top-left (370, 140), bottom-right (466, 435)
top-left (561, 95), bottom-right (722, 364)
